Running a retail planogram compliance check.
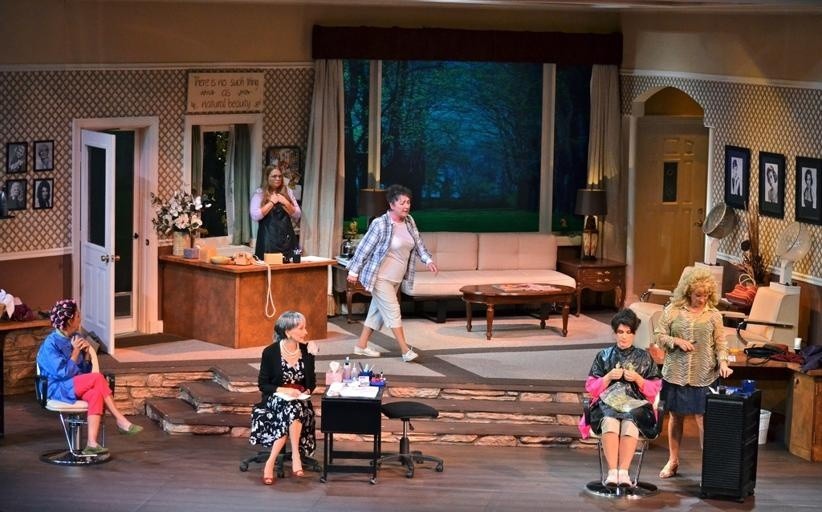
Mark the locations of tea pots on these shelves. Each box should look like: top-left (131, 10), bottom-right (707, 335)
top-left (340, 236), bottom-right (357, 257)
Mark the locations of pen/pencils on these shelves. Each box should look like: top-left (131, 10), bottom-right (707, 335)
top-left (358, 362), bottom-right (375, 374)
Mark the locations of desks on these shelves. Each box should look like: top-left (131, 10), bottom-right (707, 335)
top-left (317, 387), bottom-right (384, 487)
top-left (161, 249), bottom-right (338, 349)
top-left (714, 348), bottom-right (821, 463)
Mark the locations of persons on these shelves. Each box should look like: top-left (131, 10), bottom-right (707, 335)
top-left (248, 163), bottom-right (305, 264)
top-left (651, 260), bottom-right (737, 479)
top-left (803, 169), bottom-right (816, 208)
top-left (36, 298), bottom-right (145, 456)
top-left (577, 305), bottom-right (664, 489)
top-left (731, 157), bottom-right (742, 196)
top-left (248, 308), bottom-right (321, 485)
top-left (344, 184), bottom-right (439, 363)
top-left (765, 163), bottom-right (779, 205)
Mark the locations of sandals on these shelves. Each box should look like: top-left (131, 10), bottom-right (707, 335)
top-left (660, 461), bottom-right (679, 477)
top-left (263, 465), bottom-right (274, 484)
top-left (291, 464), bottom-right (305, 477)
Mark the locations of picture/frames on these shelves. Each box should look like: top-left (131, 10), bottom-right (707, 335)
top-left (265, 145), bottom-right (305, 207)
top-left (795, 154), bottom-right (821, 224)
top-left (1, 138), bottom-right (57, 221)
top-left (724, 142), bottom-right (750, 210)
top-left (758, 149), bottom-right (785, 219)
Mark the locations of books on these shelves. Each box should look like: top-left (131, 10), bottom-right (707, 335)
top-left (337, 384), bottom-right (380, 398)
top-left (273, 388), bottom-right (312, 405)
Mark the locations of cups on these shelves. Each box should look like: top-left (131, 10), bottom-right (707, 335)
top-left (293, 255), bottom-right (300, 262)
top-left (717, 378), bottom-right (756, 395)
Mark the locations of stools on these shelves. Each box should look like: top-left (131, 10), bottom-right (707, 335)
top-left (241, 399), bottom-right (321, 486)
top-left (381, 392), bottom-right (443, 485)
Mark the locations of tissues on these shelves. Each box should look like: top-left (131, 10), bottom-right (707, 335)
top-left (183, 244), bottom-right (201, 259)
top-left (324, 361), bottom-right (345, 386)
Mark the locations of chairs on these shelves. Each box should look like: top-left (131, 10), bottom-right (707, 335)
top-left (626, 265), bottom-right (722, 349)
top-left (35, 365), bottom-right (115, 469)
top-left (719, 285), bottom-right (801, 351)
top-left (577, 385), bottom-right (663, 502)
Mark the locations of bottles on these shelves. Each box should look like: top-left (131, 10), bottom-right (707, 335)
top-left (344, 354), bottom-right (385, 385)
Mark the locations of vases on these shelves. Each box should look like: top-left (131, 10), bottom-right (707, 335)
top-left (171, 228), bottom-right (195, 257)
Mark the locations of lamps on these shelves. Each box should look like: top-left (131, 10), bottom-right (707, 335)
top-left (574, 181), bottom-right (609, 261)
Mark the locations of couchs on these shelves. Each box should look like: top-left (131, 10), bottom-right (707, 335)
top-left (400, 229), bottom-right (575, 324)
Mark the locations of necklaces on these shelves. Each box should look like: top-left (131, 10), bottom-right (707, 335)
top-left (279, 339), bottom-right (300, 360)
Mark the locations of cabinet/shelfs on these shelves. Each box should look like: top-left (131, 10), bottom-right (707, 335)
top-left (334, 259), bottom-right (371, 325)
top-left (696, 387), bottom-right (762, 504)
top-left (561, 255), bottom-right (625, 315)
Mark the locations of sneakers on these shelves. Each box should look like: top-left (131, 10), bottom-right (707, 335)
top-left (402, 349), bottom-right (418, 362)
top-left (618, 469), bottom-right (632, 488)
top-left (605, 469), bottom-right (618, 488)
top-left (354, 343), bottom-right (380, 357)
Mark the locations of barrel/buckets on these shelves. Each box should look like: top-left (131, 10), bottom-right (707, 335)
top-left (759, 408), bottom-right (773, 446)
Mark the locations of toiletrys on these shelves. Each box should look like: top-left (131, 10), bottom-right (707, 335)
top-left (343, 357), bottom-right (351, 379)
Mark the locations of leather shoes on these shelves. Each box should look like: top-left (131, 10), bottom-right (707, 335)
top-left (82, 444), bottom-right (109, 454)
top-left (116, 424), bottom-right (144, 435)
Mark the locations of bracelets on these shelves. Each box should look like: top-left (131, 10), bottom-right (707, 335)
top-left (268, 200), bottom-right (275, 207)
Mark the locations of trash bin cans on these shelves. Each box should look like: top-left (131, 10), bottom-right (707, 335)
top-left (757, 408), bottom-right (772, 445)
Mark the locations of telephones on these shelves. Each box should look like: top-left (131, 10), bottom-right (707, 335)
top-left (232, 251), bottom-right (253, 265)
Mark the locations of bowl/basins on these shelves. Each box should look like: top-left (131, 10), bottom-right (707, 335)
top-left (212, 257), bottom-right (231, 265)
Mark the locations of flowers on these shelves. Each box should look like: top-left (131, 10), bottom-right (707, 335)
top-left (145, 185), bottom-right (215, 241)
